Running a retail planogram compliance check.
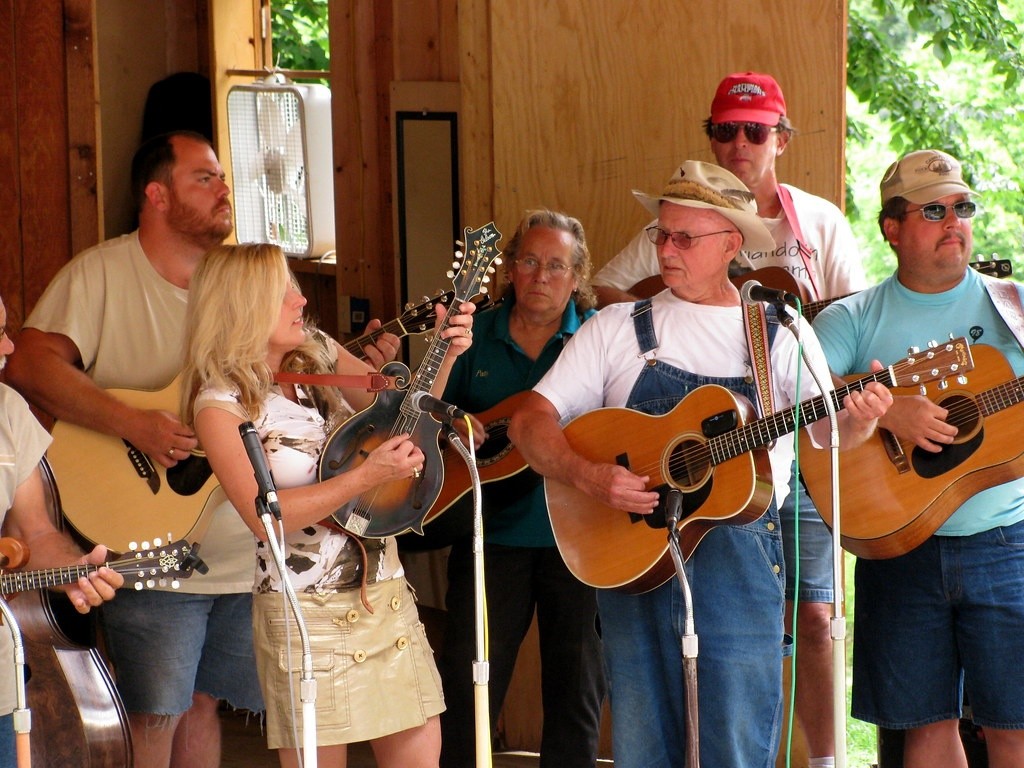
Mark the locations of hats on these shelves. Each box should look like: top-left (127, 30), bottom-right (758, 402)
top-left (631, 159), bottom-right (777, 252)
top-left (881, 150), bottom-right (981, 209)
top-left (710, 71), bottom-right (787, 127)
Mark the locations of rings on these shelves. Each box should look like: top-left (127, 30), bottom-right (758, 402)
top-left (413, 466), bottom-right (419, 478)
top-left (465, 328), bottom-right (471, 334)
top-left (166, 447), bottom-right (175, 455)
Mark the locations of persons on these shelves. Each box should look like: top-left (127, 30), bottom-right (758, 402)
top-left (6, 130), bottom-right (400, 768)
top-left (812, 150), bottom-right (1024, 768)
top-left (177, 242), bottom-right (477, 768)
top-left (507, 158), bottom-right (894, 768)
top-left (590, 70), bottom-right (868, 768)
top-left (1, 295), bottom-right (125, 768)
top-left (436, 209), bottom-right (608, 768)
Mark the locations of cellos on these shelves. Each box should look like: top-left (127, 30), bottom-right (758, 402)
top-left (7, 455), bottom-right (136, 767)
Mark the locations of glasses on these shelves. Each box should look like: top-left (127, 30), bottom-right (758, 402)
top-left (509, 253), bottom-right (574, 280)
top-left (645, 224), bottom-right (733, 250)
top-left (904, 200), bottom-right (977, 222)
top-left (710, 122), bottom-right (778, 145)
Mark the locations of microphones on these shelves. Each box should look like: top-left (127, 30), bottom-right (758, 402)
top-left (411, 391), bottom-right (466, 418)
top-left (237, 421), bottom-right (281, 521)
top-left (740, 280), bottom-right (798, 304)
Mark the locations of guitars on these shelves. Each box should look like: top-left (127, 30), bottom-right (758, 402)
top-left (400, 384), bottom-right (537, 551)
top-left (0, 534), bottom-right (213, 596)
top-left (313, 221), bottom-right (504, 539)
top-left (43, 287), bottom-right (495, 560)
top-left (626, 252), bottom-right (1014, 325)
top-left (793, 342), bottom-right (1024, 560)
top-left (538, 332), bottom-right (977, 598)
